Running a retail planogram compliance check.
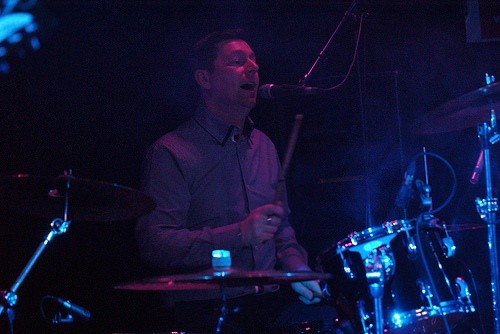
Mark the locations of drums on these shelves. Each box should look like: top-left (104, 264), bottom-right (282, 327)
top-left (317, 214), bottom-right (475, 334)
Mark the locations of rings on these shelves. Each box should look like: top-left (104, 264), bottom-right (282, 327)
top-left (267, 216), bottom-right (272, 224)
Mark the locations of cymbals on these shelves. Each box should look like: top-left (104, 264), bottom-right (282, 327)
top-left (156, 267), bottom-right (335, 282)
top-left (408, 82), bottom-right (500, 135)
top-left (112, 276), bottom-right (219, 290)
top-left (0, 174), bottom-right (157, 223)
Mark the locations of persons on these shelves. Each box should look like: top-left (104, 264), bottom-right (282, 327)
top-left (133, 28), bottom-right (323, 334)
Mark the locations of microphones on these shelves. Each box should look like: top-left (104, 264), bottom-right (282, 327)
top-left (395, 159), bottom-right (417, 208)
top-left (257, 84), bottom-right (319, 98)
top-left (53, 297), bottom-right (90, 320)
